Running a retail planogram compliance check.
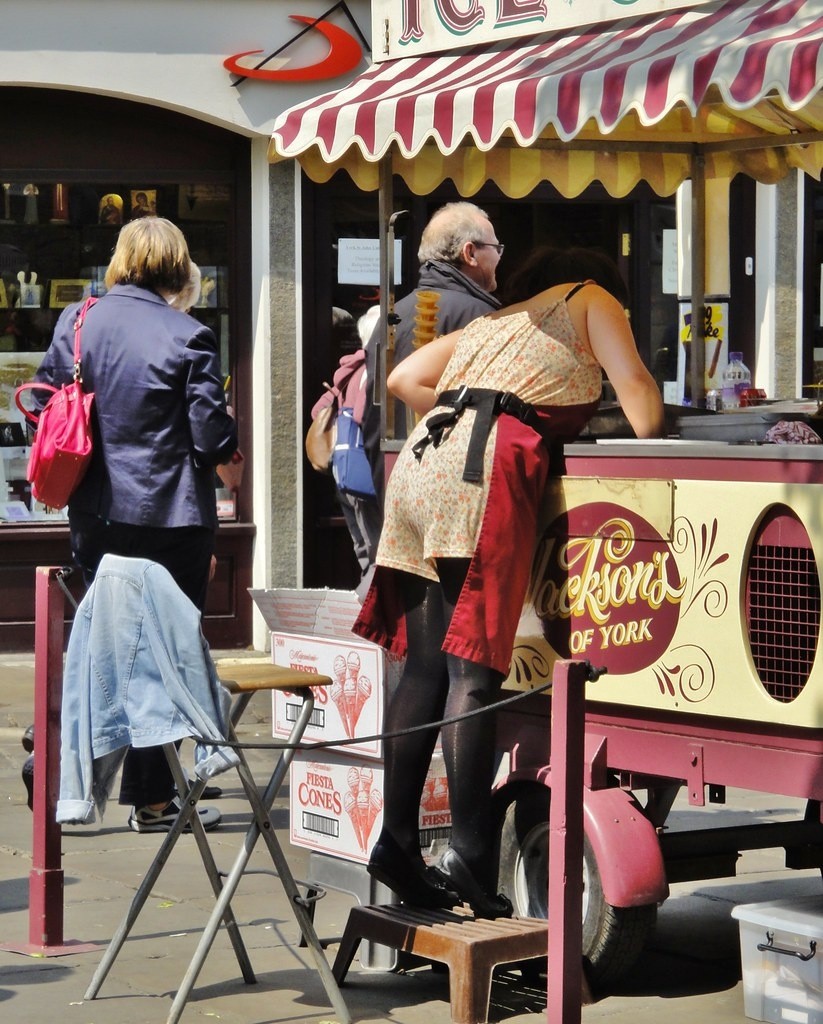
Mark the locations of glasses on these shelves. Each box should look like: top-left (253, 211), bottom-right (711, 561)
top-left (474, 241), bottom-right (505, 255)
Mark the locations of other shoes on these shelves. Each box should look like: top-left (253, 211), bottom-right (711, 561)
top-left (367, 837), bottom-right (463, 909)
top-left (437, 849), bottom-right (513, 918)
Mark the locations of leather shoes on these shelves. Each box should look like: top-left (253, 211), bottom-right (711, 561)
top-left (177, 779), bottom-right (222, 798)
top-left (22, 725), bottom-right (35, 752)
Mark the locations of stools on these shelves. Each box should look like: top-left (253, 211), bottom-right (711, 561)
top-left (298, 852), bottom-right (399, 971)
top-left (57, 553), bottom-right (352, 1024)
top-left (332, 905), bottom-right (593, 1024)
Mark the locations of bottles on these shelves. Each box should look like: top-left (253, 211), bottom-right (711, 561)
top-left (721, 351), bottom-right (751, 411)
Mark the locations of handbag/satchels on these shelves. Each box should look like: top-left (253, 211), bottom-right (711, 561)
top-left (15, 297), bottom-right (97, 509)
top-left (304, 378), bottom-right (352, 475)
top-left (332, 408), bottom-right (375, 498)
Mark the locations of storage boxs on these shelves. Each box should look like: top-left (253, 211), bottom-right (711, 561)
top-left (289, 745), bottom-right (510, 870)
top-left (246, 585), bottom-right (444, 759)
top-left (731, 896), bottom-right (823, 1024)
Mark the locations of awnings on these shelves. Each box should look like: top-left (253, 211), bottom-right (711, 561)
top-left (267, 2), bottom-right (823, 199)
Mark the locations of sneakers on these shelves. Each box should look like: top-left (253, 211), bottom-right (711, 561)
top-left (23, 754), bottom-right (34, 810)
top-left (129, 795), bottom-right (221, 832)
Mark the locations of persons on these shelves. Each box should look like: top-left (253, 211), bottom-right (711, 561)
top-left (306, 199), bottom-right (505, 580)
top-left (349, 243), bottom-right (663, 921)
top-left (26, 214), bottom-right (240, 834)
